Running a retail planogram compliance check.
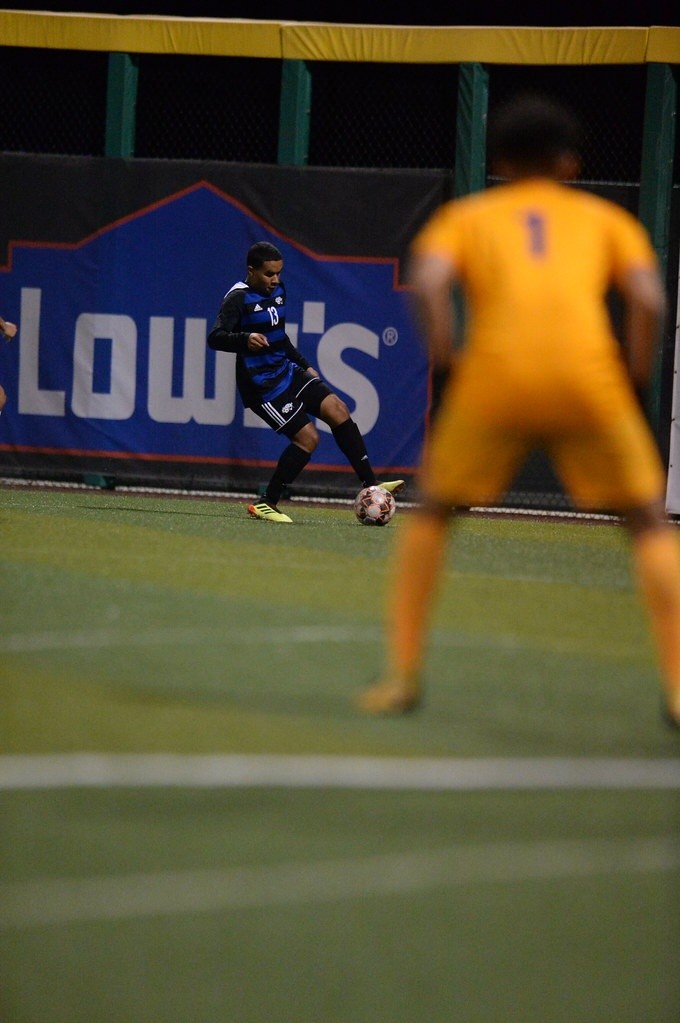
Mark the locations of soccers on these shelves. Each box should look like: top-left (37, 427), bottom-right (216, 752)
top-left (354, 486), bottom-right (395, 527)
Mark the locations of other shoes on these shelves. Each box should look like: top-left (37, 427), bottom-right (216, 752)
top-left (358, 686), bottom-right (418, 714)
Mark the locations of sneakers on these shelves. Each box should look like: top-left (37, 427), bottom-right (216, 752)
top-left (377, 480), bottom-right (405, 497)
top-left (249, 500), bottom-right (292, 522)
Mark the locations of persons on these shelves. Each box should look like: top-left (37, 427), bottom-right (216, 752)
top-left (0, 314), bottom-right (16, 413)
top-left (354, 95), bottom-right (679, 732)
top-left (206, 242), bottom-right (406, 523)
